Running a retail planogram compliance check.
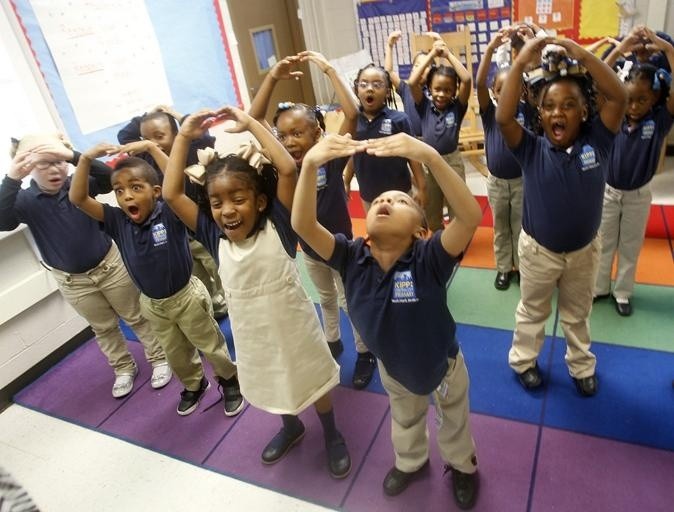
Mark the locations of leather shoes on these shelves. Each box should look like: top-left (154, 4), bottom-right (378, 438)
top-left (612, 292), bottom-right (631, 316)
top-left (324, 434), bottom-right (352, 478)
top-left (574, 373), bottom-right (597, 396)
top-left (261, 416), bottom-right (305, 463)
top-left (443, 464), bottom-right (479, 508)
top-left (517, 361), bottom-right (541, 387)
top-left (384, 461), bottom-right (428, 495)
top-left (494, 270), bottom-right (511, 290)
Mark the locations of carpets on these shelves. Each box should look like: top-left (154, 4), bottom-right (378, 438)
top-left (11, 187), bottom-right (674, 511)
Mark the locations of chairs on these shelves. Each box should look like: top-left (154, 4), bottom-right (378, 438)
top-left (413, 33), bottom-right (493, 183)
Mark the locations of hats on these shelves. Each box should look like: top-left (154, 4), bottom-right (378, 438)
top-left (17, 134), bottom-right (71, 161)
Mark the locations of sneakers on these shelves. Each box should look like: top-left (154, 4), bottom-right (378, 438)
top-left (150, 362), bottom-right (172, 389)
top-left (353, 356), bottom-right (377, 386)
top-left (328, 340), bottom-right (343, 356)
top-left (112, 368), bottom-right (138, 397)
top-left (176, 377), bottom-right (212, 416)
top-left (202, 377), bottom-right (244, 416)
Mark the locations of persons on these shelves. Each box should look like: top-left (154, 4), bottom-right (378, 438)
top-left (67, 138), bottom-right (245, 419)
top-left (116, 18), bottom-right (673, 390)
top-left (493, 33), bottom-right (627, 399)
top-left (287, 125), bottom-right (483, 510)
top-left (160, 105), bottom-right (356, 480)
top-left (0, 129), bottom-right (174, 399)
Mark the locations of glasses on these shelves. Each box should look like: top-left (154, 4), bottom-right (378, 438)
top-left (35, 160), bottom-right (67, 168)
top-left (357, 81), bottom-right (389, 88)
